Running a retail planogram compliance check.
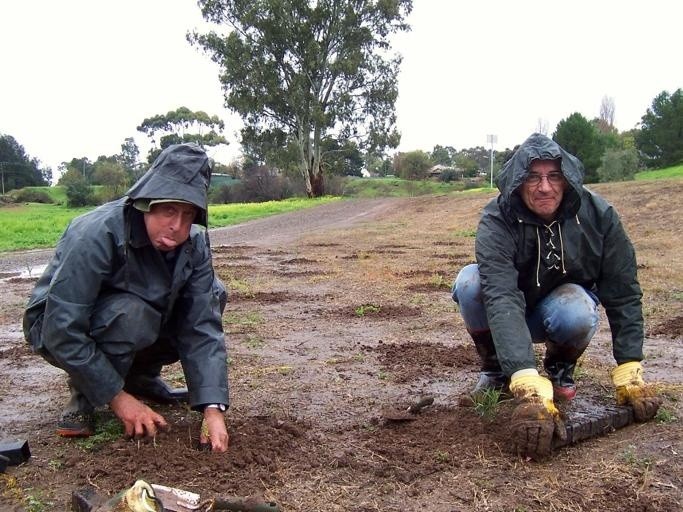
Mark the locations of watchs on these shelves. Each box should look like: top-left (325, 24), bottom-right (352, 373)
top-left (204, 402), bottom-right (226, 412)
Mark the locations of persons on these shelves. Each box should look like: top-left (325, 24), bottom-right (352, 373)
top-left (447, 132), bottom-right (663, 461)
top-left (19, 141), bottom-right (233, 455)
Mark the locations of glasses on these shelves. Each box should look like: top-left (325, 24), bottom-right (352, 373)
top-left (524, 171), bottom-right (564, 188)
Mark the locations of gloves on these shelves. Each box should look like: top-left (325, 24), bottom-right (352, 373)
top-left (611, 362), bottom-right (661, 421)
top-left (509, 373), bottom-right (569, 458)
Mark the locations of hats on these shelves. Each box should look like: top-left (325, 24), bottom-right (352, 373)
top-left (133, 197), bottom-right (191, 212)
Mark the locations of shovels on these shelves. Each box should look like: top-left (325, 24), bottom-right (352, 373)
top-left (384, 397), bottom-right (434, 420)
top-left (150, 484), bottom-right (280, 512)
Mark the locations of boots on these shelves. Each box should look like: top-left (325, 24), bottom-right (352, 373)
top-left (462, 327), bottom-right (510, 407)
top-left (543, 341), bottom-right (586, 402)
top-left (127, 349), bottom-right (189, 403)
top-left (56, 376), bottom-right (94, 438)
